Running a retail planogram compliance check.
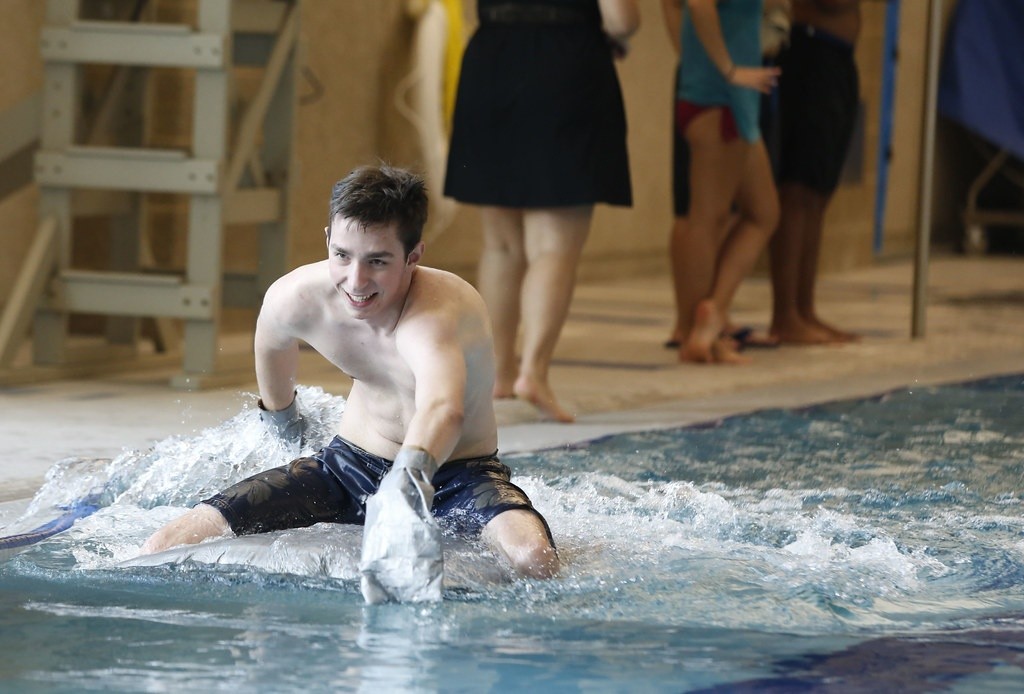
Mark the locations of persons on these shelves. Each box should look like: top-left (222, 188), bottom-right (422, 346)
top-left (134, 162), bottom-right (558, 603)
top-left (760, 0), bottom-right (861, 345)
top-left (441, 0), bottom-right (641, 422)
top-left (656, 0), bottom-right (780, 368)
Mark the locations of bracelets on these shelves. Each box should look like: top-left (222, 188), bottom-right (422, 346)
top-left (724, 62), bottom-right (737, 82)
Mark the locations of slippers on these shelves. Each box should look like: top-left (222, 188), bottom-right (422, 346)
top-left (734, 328), bottom-right (778, 347)
top-left (668, 342), bottom-right (746, 351)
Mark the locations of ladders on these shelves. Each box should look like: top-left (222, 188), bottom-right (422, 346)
top-left (23, 0), bottom-right (301, 382)
